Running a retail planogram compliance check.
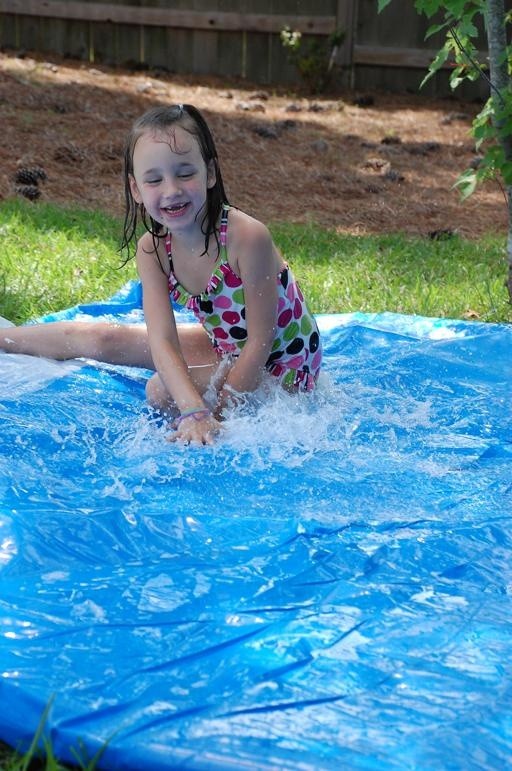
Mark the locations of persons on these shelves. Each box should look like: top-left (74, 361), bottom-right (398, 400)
top-left (1, 104), bottom-right (323, 449)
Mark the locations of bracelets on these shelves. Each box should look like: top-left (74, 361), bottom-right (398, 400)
top-left (171, 406), bottom-right (211, 430)
